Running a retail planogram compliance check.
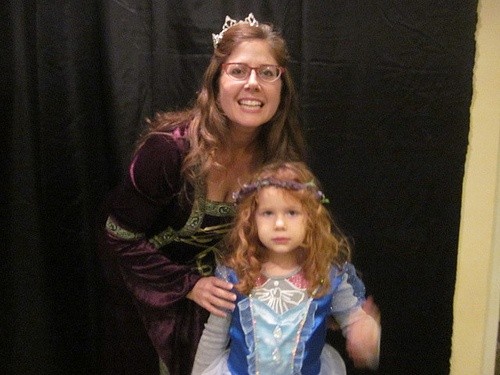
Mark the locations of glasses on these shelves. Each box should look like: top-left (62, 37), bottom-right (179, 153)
top-left (224, 62), bottom-right (282, 82)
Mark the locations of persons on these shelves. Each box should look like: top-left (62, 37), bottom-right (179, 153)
top-left (104, 22), bottom-right (381, 375)
top-left (191, 161), bottom-right (381, 375)
